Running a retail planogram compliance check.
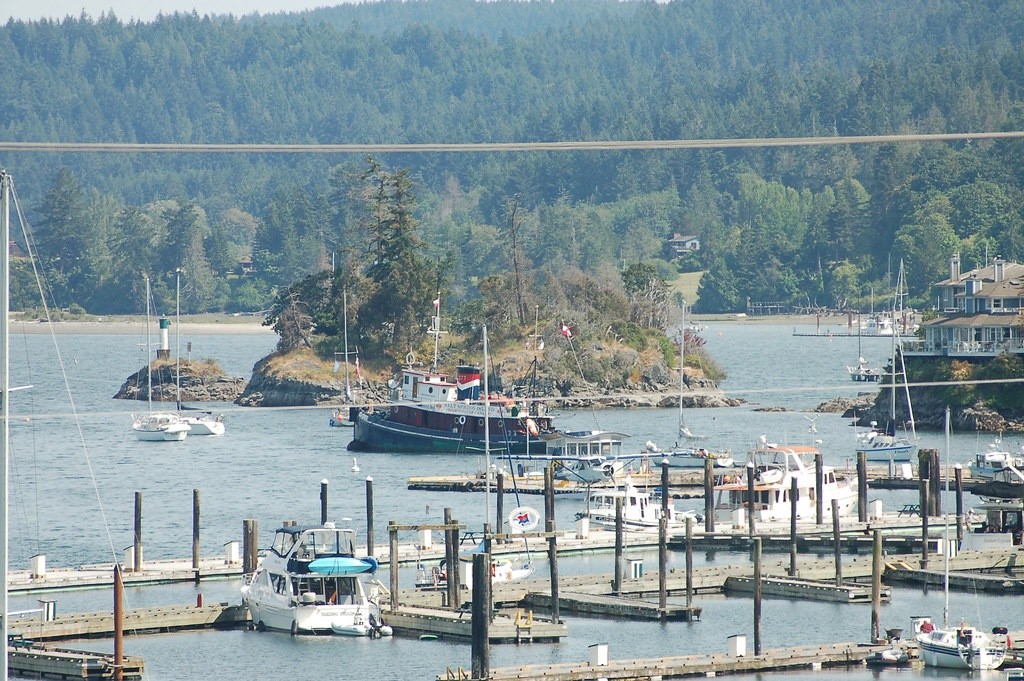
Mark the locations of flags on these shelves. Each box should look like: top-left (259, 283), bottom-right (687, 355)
top-left (432, 298), bottom-right (440, 307)
top-left (561, 325), bottom-right (571, 337)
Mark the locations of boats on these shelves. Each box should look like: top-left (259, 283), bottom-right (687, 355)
top-left (847, 299), bottom-right (880, 380)
top-left (522, 430), bottom-right (628, 477)
top-left (703, 437), bottom-right (872, 526)
top-left (863, 647), bottom-right (911, 663)
top-left (240, 525), bottom-right (383, 638)
top-left (574, 483), bottom-right (702, 531)
top-left (969, 430), bottom-right (1024, 482)
top-left (330, 284), bottom-right (631, 459)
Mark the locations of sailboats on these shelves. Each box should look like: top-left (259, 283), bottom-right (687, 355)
top-left (643, 299), bottom-right (734, 469)
top-left (132, 268), bottom-right (224, 440)
top-left (414, 325), bottom-right (539, 590)
top-left (856, 309), bottom-right (920, 463)
top-left (917, 404), bottom-right (1006, 671)
